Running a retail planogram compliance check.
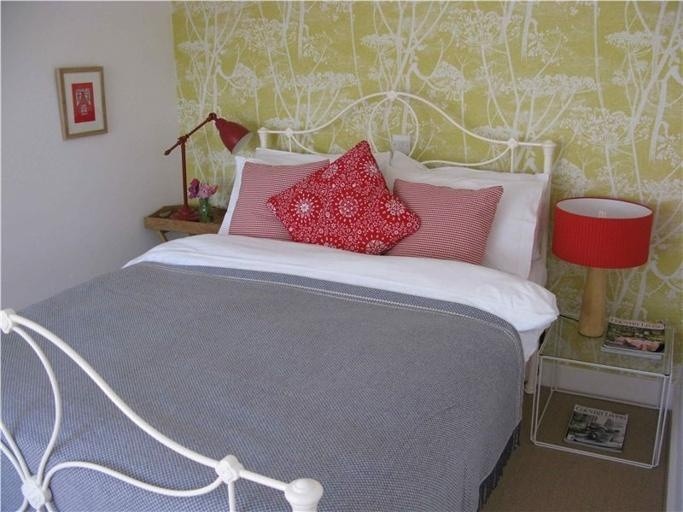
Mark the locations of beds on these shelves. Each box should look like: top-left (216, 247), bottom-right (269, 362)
top-left (0, 90), bottom-right (557, 511)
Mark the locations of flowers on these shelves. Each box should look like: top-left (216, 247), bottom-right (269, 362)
top-left (188, 179), bottom-right (218, 199)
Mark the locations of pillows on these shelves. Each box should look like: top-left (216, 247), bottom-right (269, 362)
top-left (388, 176), bottom-right (507, 266)
top-left (388, 149), bottom-right (549, 278)
top-left (228, 156), bottom-right (335, 242)
top-left (217, 150), bottom-right (394, 249)
top-left (263, 140), bottom-right (423, 256)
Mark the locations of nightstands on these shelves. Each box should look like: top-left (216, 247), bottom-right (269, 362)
top-left (145, 205), bottom-right (226, 242)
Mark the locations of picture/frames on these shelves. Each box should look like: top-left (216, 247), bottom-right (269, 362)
top-left (55, 66), bottom-right (108, 141)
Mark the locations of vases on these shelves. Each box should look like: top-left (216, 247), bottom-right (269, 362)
top-left (199, 199), bottom-right (212, 223)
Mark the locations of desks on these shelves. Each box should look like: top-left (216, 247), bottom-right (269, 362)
top-left (529, 313), bottom-right (675, 470)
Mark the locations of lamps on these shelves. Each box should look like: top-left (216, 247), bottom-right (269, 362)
top-left (164, 112), bottom-right (253, 214)
top-left (553, 197), bottom-right (653, 338)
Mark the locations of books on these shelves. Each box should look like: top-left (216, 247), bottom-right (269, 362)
top-left (563, 404), bottom-right (629, 453)
top-left (600, 314), bottom-right (666, 360)
